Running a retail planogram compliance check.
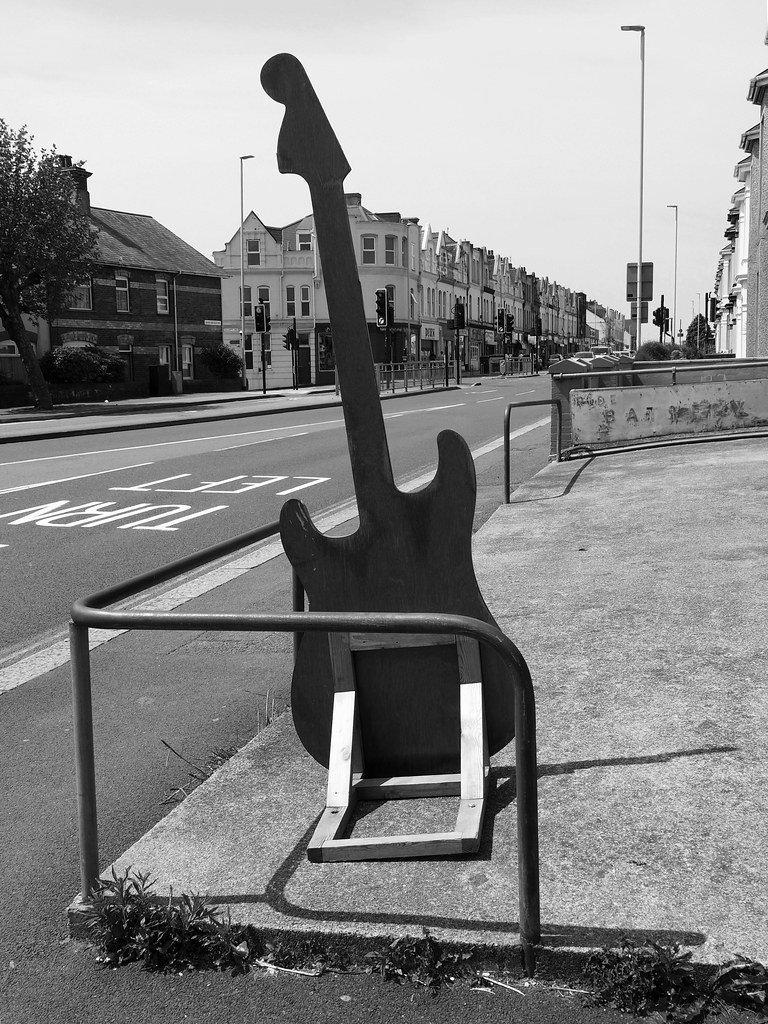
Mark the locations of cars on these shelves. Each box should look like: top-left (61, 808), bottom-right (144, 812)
top-left (573, 344), bottom-right (636, 362)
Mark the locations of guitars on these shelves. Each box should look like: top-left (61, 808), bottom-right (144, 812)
top-left (258, 52), bottom-right (519, 775)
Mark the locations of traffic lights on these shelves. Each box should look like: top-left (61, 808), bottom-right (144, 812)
top-left (375, 288), bottom-right (387, 327)
top-left (282, 335), bottom-right (289, 350)
top-left (255, 305), bottom-right (265, 333)
top-left (266, 317), bottom-right (270, 332)
top-left (652, 307), bottom-right (662, 327)
top-left (506, 313), bottom-right (514, 333)
top-left (498, 309), bottom-right (504, 333)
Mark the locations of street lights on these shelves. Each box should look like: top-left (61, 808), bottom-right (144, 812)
top-left (621, 26), bottom-right (645, 356)
top-left (696, 292), bottom-right (700, 350)
top-left (667, 205), bottom-right (678, 342)
top-left (240, 155), bottom-right (254, 391)
